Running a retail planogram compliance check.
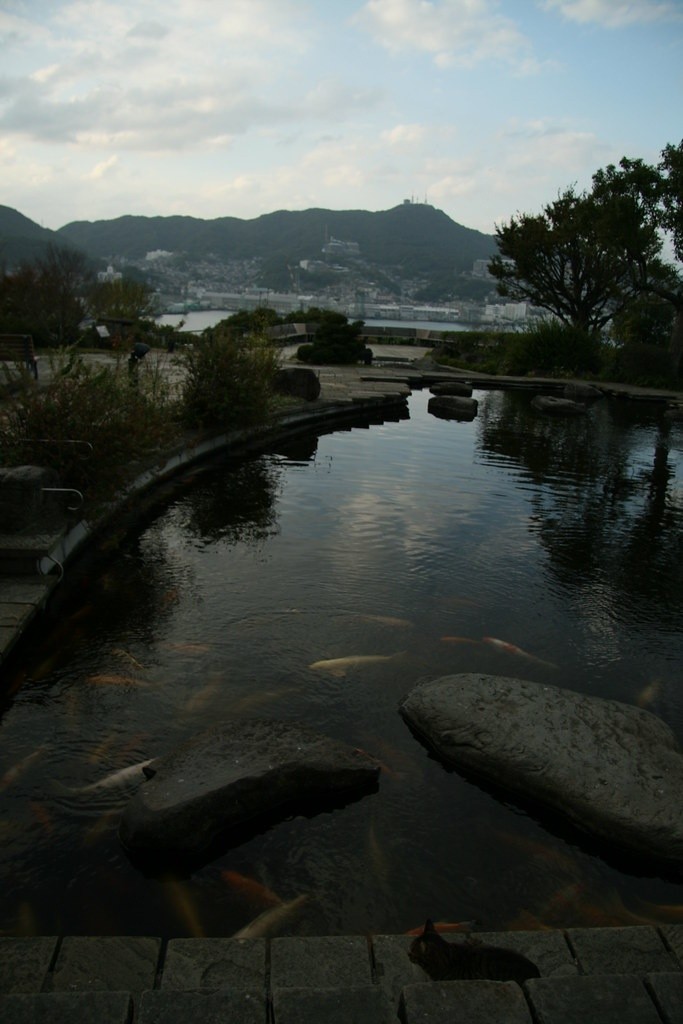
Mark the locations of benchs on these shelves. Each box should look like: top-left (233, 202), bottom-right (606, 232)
top-left (0, 334), bottom-right (40, 379)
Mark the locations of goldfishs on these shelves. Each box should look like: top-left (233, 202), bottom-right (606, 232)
top-left (0, 598), bottom-right (683, 973)
